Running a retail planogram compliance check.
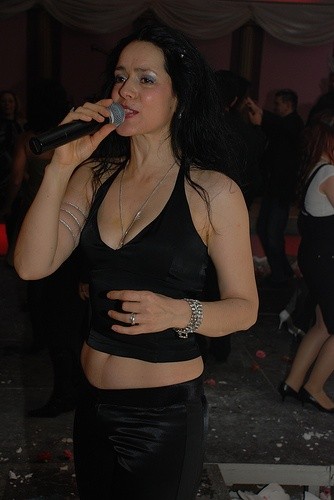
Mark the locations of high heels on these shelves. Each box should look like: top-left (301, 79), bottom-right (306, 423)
top-left (278, 380), bottom-right (334, 414)
top-left (278, 309), bottom-right (302, 337)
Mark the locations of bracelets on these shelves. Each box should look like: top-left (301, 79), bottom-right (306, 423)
top-left (175, 298), bottom-right (203, 340)
top-left (57, 201), bottom-right (87, 246)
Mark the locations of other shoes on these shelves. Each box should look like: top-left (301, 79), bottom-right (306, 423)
top-left (261, 265), bottom-right (295, 285)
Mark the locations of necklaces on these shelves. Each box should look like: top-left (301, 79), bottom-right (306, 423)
top-left (120, 158), bottom-right (176, 246)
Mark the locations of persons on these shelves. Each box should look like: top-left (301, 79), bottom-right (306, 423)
top-left (0, 44), bottom-right (333, 415)
top-left (14, 23), bottom-right (258, 500)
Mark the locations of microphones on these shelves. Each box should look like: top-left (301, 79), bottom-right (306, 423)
top-left (28, 104), bottom-right (125, 154)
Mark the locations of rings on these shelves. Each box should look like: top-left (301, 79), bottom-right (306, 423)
top-left (130, 313), bottom-right (136, 324)
